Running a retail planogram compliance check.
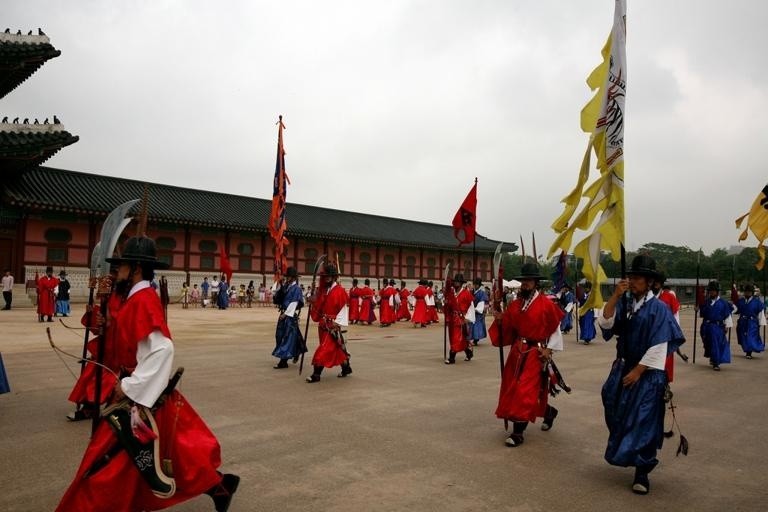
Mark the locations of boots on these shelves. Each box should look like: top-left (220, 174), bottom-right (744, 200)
top-left (305, 364), bottom-right (325, 384)
top-left (505, 417), bottom-right (529, 447)
top-left (631, 461), bottom-right (651, 494)
top-left (337, 357), bottom-right (353, 378)
top-left (541, 404), bottom-right (559, 431)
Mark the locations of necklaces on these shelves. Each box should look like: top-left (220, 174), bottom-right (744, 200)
top-left (628, 293), bottom-right (648, 315)
top-left (520, 291), bottom-right (536, 313)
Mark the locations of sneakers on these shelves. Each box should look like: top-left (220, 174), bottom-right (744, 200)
top-left (272, 361), bottom-right (289, 369)
top-left (66, 405), bottom-right (100, 422)
top-left (463, 350), bottom-right (473, 361)
top-left (709, 360), bottom-right (721, 371)
top-left (584, 336), bottom-right (591, 345)
top-left (444, 356), bottom-right (456, 365)
top-left (207, 468), bottom-right (241, 512)
top-left (745, 351), bottom-right (753, 359)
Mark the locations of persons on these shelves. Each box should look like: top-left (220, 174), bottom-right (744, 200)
top-left (36, 266), bottom-right (59, 322)
top-left (306, 265), bottom-right (353, 382)
top-left (271, 267), bottom-right (308, 369)
top-left (57, 237), bottom-right (242, 511)
top-left (54, 269), bottom-right (71, 317)
top-left (653, 270), bottom-right (681, 392)
top-left (151, 272), bottom-right (169, 307)
top-left (0, 270), bottom-right (14, 310)
top-left (489, 265), bottom-right (564, 445)
top-left (67, 276), bottom-right (116, 421)
top-left (181, 276), bottom-right (318, 309)
top-left (731, 284), bottom-right (767, 359)
top-left (597, 253), bottom-right (686, 492)
top-left (503, 281), bottom-right (597, 344)
top-left (694, 281), bottom-right (733, 371)
top-left (349, 277), bottom-right (439, 328)
top-left (442, 273), bottom-right (477, 364)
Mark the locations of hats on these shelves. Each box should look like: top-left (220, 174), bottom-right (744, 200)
top-left (512, 262), bottom-right (548, 280)
top-left (352, 278), bottom-right (434, 287)
top-left (704, 281), bottom-right (724, 289)
top-left (623, 252), bottom-right (669, 282)
top-left (282, 267), bottom-right (303, 278)
top-left (740, 284), bottom-right (758, 294)
top-left (451, 273), bottom-right (484, 287)
top-left (317, 263), bottom-right (342, 277)
top-left (560, 283), bottom-right (574, 290)
top-left (45, 265), bottom-right (68, 276)
top-left (103, 232), bottom-right (174, 267)
